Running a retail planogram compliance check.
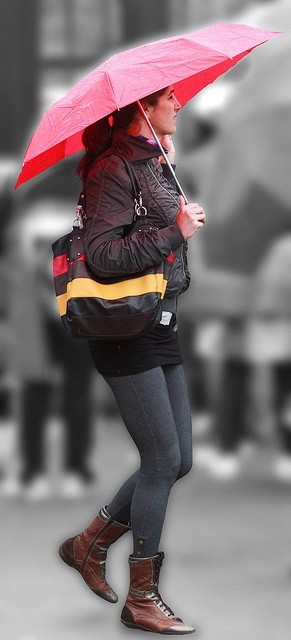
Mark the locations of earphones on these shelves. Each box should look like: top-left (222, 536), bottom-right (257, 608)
top-left (145, 111), bottom-right (150, 119)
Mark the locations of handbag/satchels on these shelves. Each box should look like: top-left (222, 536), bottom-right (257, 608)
top-left (52, 150), bottom-right (174, 340)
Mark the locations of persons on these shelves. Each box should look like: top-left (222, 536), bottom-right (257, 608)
top-left (57, 82), bottom-right (207, 638)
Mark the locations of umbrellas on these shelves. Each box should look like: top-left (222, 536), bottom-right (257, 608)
top-left (14, 21), bottom-right (284, 208)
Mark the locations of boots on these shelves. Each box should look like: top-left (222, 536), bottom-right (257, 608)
top-left (121, 551), bottom-right (195, 634)
top-left (59, 505), bottom-right (132, 603)
